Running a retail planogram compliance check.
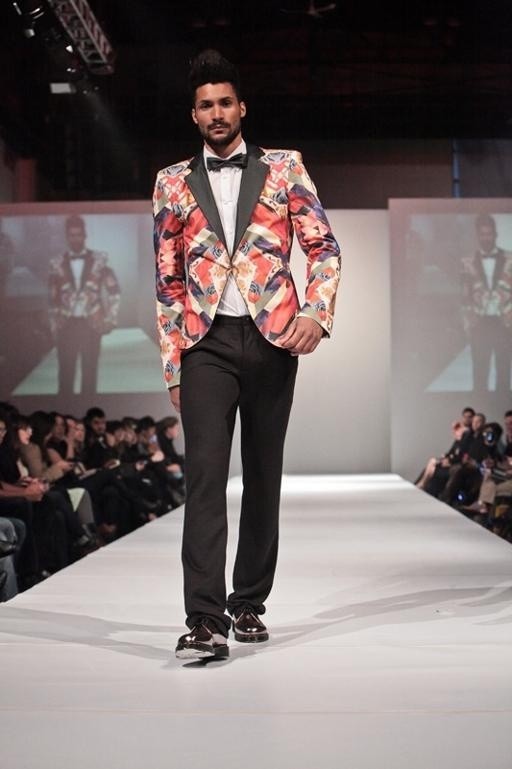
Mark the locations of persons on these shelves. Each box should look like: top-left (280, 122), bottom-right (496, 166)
top-left (461, 213), bottom-right (512, 392)
top-left (0, 401), bottom-right (185, 602)
top-left (152, 62), bottom-right (341, 662)
top-left (45, 214), bottom-right (121, 396)
top-left (412, 405), bottom-right (512, 542)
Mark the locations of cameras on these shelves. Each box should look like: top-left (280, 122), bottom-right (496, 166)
top-left (487, 432), bottom-right (495, 442)
top-left (66, 456), bottom-right (83, 463)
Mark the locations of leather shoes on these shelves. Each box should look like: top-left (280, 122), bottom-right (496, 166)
top-left (174, 616), bottom-right (232, 660)
top-left (464, 501), bottom-right (487, 514)
top-left (232, 606), bottom-right (269, 643)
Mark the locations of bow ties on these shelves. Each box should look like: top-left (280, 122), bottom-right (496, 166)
top-left (70, 255), bottom-right (86, 261)
top-left (482, 254), bottom-right (496, 259)
top-left (207, 152), bottom-right (247, 172)
